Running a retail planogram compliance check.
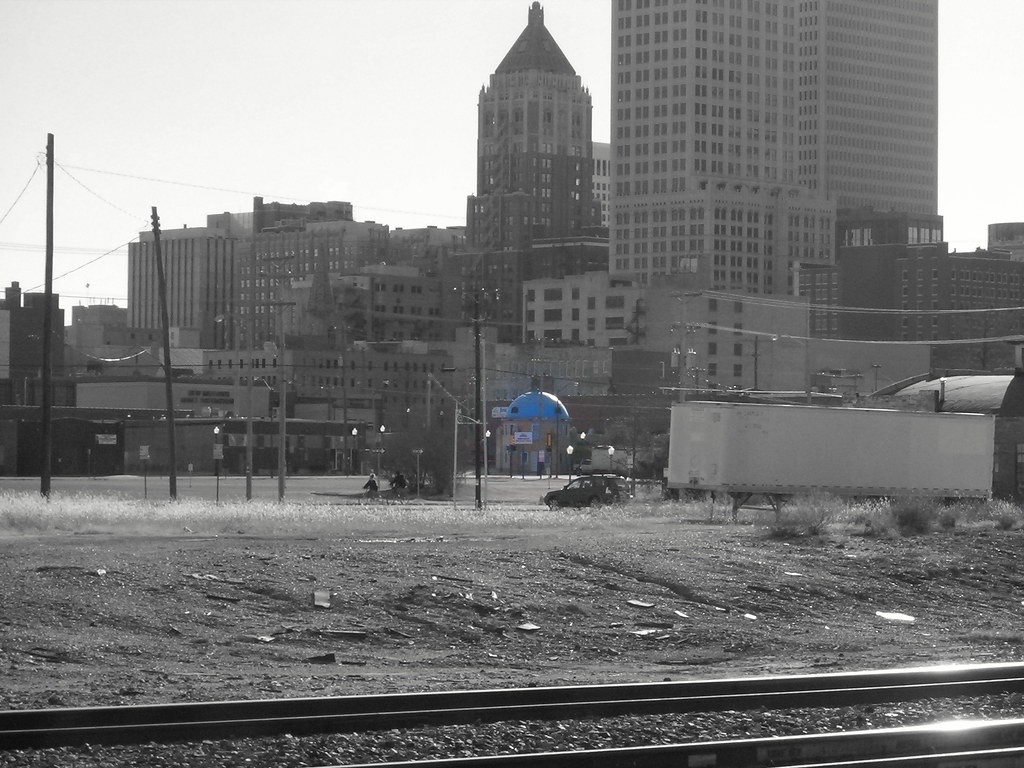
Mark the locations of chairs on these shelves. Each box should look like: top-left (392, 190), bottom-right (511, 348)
top-left (584, 483), bottom-right (591, 488)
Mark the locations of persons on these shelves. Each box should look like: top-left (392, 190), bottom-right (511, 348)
top-left (364, 473), bottom-right (379, 501)
top-left (389, 470), bottom-right (408, 501)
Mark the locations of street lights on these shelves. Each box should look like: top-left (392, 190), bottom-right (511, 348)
top-left (872, 364), bottom-right (882, 390)
top-left (556, 382), bottom-right (578, 478)
top-left (608, 446), bottom-right (615, 473)
top-left (410, 448), bottom-right (424, 500)
top-left (579, 432), bottom-right (586, 473)
top-left (380, 425), bottom-right (386, 447)
top-left (214, 426), bottom-right (223, 503)
top-left (214, 312), bottom-right (253, 501)
top-left (372, 447), bottom-right (386, 491)
top-left (567, 445), bottom-right (574, 484)
top-left (351, 428), bottom-right (358, 475)
top-left (253, 375), bottom-right (274, 479)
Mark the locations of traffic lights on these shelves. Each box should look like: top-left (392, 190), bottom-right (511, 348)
top-left (506, 443), bottom-right (516, 451)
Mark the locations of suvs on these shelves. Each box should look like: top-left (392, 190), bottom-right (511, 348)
top-left (544, 474), bottom-right (631, 511)
